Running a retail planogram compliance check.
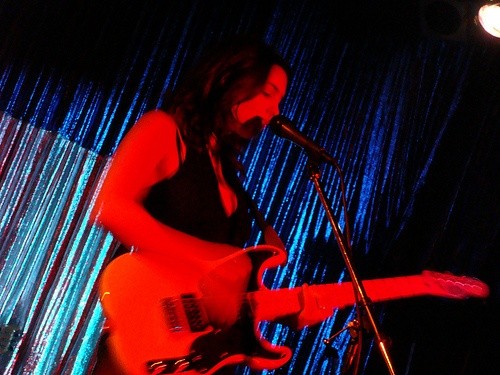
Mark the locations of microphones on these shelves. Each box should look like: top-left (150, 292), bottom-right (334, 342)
top-left (271, 115), bottom-right (334, 163)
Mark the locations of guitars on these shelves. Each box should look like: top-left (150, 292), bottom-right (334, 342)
top-left (99, 244), bottom-right (490, 375)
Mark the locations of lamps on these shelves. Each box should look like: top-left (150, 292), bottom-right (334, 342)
top-left (478, 0), bottom-right (500, 40)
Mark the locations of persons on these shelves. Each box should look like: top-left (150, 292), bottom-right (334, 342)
top-left (90, 36), bottom-right (293, 374)
